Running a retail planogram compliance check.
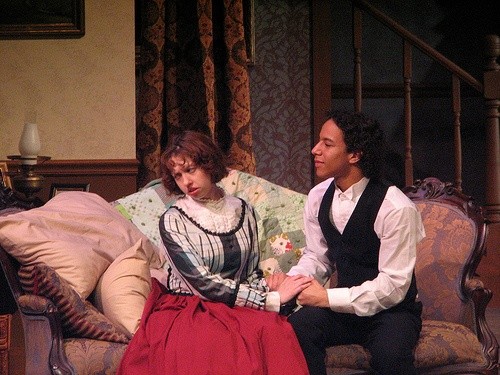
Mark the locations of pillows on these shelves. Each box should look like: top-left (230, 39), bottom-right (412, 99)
top-left (0, 191), bottom-right (167, 302)
top-left (95, 238), bottom-right (152, 336)
top-left (18, 264), bottom-right (129, 344)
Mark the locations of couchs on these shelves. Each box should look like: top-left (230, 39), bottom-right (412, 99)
top-left (0, 175), bottom-right (500, 375)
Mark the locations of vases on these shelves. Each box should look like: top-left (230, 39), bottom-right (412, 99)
top-left (18, 123), bottom-right (41, 159)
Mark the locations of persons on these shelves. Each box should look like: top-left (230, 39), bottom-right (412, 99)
top-left (116, 131), bottom-right (314, 375)
top-left (265, 110), bottom-right (426, 375)
